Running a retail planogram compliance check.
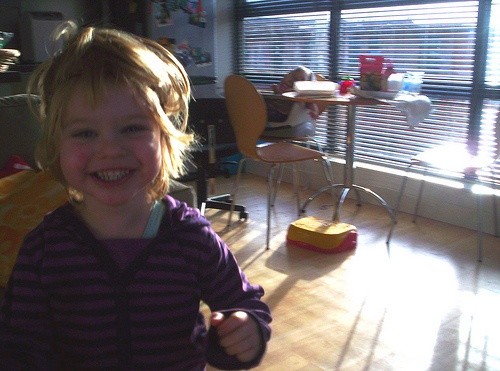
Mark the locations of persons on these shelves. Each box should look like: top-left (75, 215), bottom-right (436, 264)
top-left (267, 66), bottom-right (319, 123)
top-left (0, 21), bottom-right (273, 370)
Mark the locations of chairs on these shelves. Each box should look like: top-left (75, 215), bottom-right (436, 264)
top-left (261, 65), bottom-right (334, 218)
top-left (223, 75), bottom-right (334, 250)
top-left (386, 105), bottom-right (500, 262)
top-left (175, 98), bottom-right (248, 223)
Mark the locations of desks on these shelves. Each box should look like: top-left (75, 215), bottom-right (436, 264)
top-left (282, 89), bottom-right (407, 225)
